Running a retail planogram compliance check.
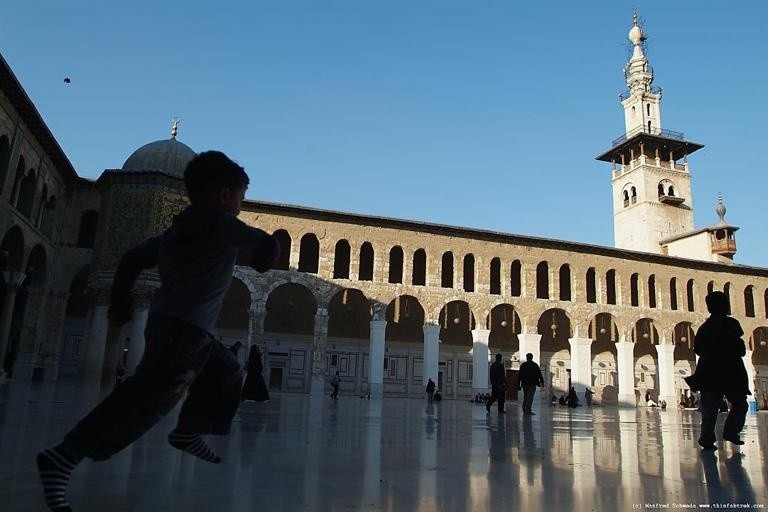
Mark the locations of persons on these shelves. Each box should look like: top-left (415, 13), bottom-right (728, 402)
top-left (484, 393), bottom-right (491, 404)
top-left (329, 370), bottom-right (343, 400)
top-left (330, 401), bottom-right (338, 435)
top-left (433, 390), bottom-right (441, 402)
top-left (482, 415), bottom-right (515, 493)
top-left (35, 148), bottom-right (285, 511)
top-left (682, 290), bottom-right (753, 450)
top-left (696, 450), bottom-right (759, 512)
top-left (424, 404), bottom-right (435, 442)
top-left (424, 377), bottom-right (435, 403)
top-left (645, 389), bottom-right (767, 415)
top-left (240, 344), bottom-right (270, 403)
top-left (480, 393), bottom-right (486, 404)
top-left (114, 360), bottom-right (126, 386)
top-left (517, 353), bottom-right (545, 416)
top-left (474, 393), bottom-right (482, 403)
top-left (558, 395), bottom-right (566, 405)
top-left (485, 353), bottom-right (508, 416)
top-left (516, 415), bottom-right (543, 489)
top-left (566, 386), bottom-right (577, 409)
top-left (584, 388), bottom-right (595, 407)
top-left (228, 339), bottom-right (242, 358)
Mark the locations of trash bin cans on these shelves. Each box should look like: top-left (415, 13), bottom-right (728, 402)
top-left (750, 401), bottom-right (757, 412)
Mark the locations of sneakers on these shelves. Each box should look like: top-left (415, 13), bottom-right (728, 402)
top-left (699, 438), bottom-right (717, 450)
top-left (721, 433), bottom-right (744, 445)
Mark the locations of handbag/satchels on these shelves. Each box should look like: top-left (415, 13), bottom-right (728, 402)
top-left (247, 359), bottom-right (262, 377)
